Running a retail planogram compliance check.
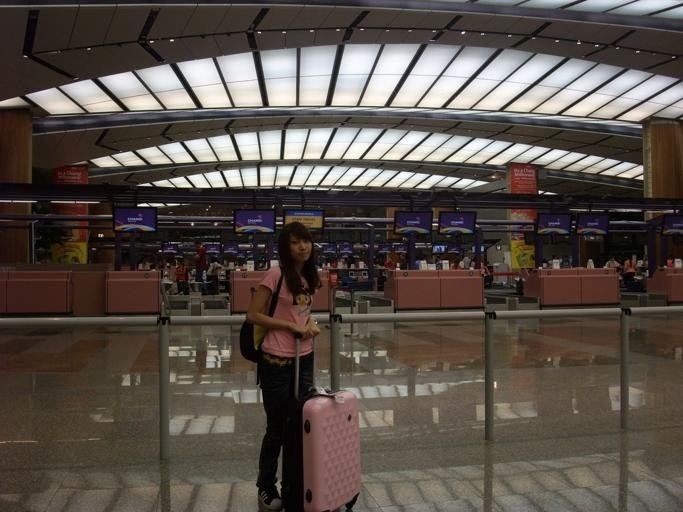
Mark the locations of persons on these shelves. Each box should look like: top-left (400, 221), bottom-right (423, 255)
top-left (602, 253), bottom-right (682, 292)
top-left (120, 239), bottom-right (247, 295)
top-left (244, 220), bottom-right (321, 512)
top-left (313, 247), bottom-right (493, 288)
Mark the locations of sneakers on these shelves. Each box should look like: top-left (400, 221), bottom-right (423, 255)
top-left (258, 484), bottom-right (282, 511)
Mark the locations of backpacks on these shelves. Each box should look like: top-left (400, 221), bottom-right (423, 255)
top-left (239, 264), bottom-right (285, 363)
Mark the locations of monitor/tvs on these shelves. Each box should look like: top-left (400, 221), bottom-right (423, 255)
top-left (283, 209), bottom-right (326, 234)
top-left (393, 210), bottom-right (433, 235)
top-left (234, 208), bottom-right (276, 234)
top-left (319, 242), bottom-right (488, 259)
top-left (437, 211), bottom-right (477, 235)
top-left (660, 213), bottom-right (683, 237)
top-left (574, 212), bottom-right (610, 237)
top-left (534, 212), bottom-right (572, 236)
top-left (163, 244), bottom-right (248, 259)
top-left (112, 205), bottom-right (158, 233)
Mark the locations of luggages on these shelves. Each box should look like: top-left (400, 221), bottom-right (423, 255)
top-left (282, 332), bottom-right (360, 512)
